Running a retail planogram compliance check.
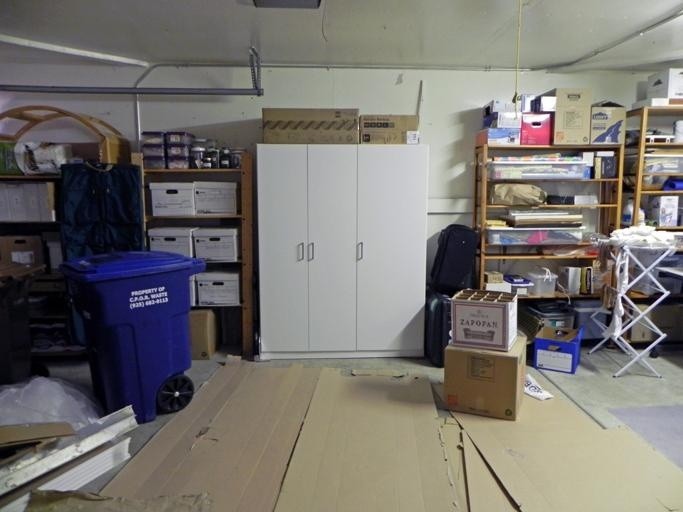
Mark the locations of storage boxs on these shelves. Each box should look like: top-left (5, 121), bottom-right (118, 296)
top-left (0, 236), bottom-right (44, 267)
top-left (444, 337), bottom-right (527, 421)
top-left (647, 68), bottom-right (683, 105)
top-left (534, 326), bottom-right (583, 375)
top-left (451, 287), bottom-right (518, 352)
top-left (529, 301), bottom-right (607, 340)
top-left (262, 107), bottom-right (359, 143)
top-left (0, 182), bottom-right (55, 222)
top-left (149, 181), bottom-right (241, 358)
top-left (142, 130), bottom-right (194, 168)
top-left (360, 79), bottom-right (423, 144)
top-left (643, 193), bottom-right (679, 226)
top-left (474, 88), bottom-right (625, 144)
top-left (47, 232), bottom-right (63, 269)
top-left (630, 302), bottom-right (683, 343)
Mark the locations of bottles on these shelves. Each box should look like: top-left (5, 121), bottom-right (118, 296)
top-left (623, 197), bottom-right (646, 225)
top-left (192, 139), bottom-right (239, 169)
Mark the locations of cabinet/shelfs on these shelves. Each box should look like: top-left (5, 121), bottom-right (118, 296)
top-left (256, 143), bottom-right (358, 353)
top-left (137, 152), bottom-right (252, 359)
top-left (473, 139), bottom-right (624, 301)
top-left (610, 104), bottom-right (683, 229)
top-left (590, 237), bottom-right (675, 378)
top-left (358, 144), bottom-right (427, 352)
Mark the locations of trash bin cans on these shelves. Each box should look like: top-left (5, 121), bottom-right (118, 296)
top-left (57, 250), bottom-right (206, 424)
